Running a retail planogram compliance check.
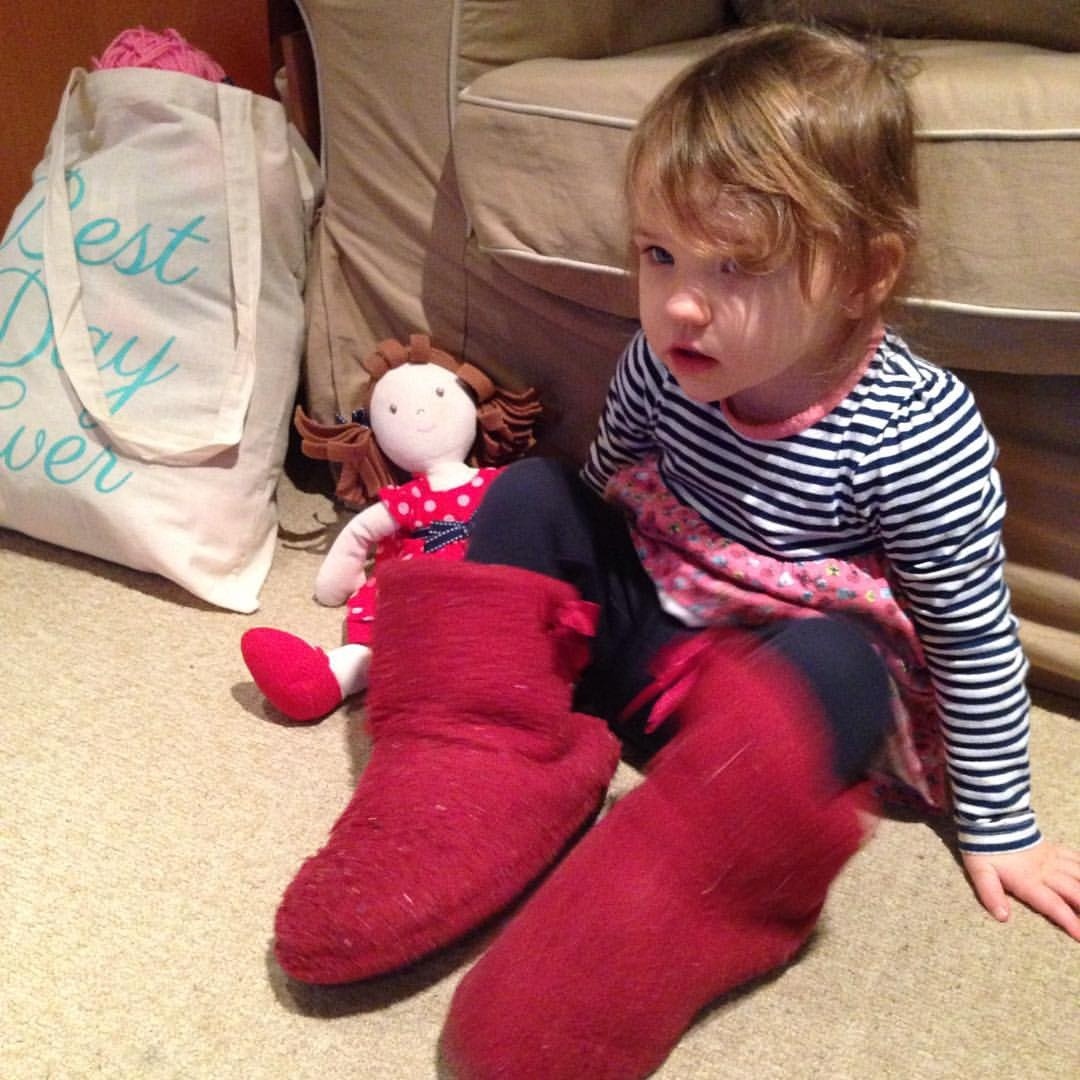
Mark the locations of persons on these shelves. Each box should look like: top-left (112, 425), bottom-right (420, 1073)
top-left (275, 25), bottom-right (1080, 1080)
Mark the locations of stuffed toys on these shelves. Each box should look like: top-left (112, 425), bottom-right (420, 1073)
top-left (245, 334), bottom-right (543, 722)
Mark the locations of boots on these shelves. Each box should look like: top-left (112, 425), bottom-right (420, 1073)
top-left (275, 560), bottom-right (624, 985)
top-left (429, 622), bottom-right (876, 1080)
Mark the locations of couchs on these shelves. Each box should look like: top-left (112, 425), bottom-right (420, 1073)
top-left (293, 0), bottom-right (1079, 699)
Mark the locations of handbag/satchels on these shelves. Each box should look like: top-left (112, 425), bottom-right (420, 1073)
top-left (0, 62), bottom-right (324, 614)
